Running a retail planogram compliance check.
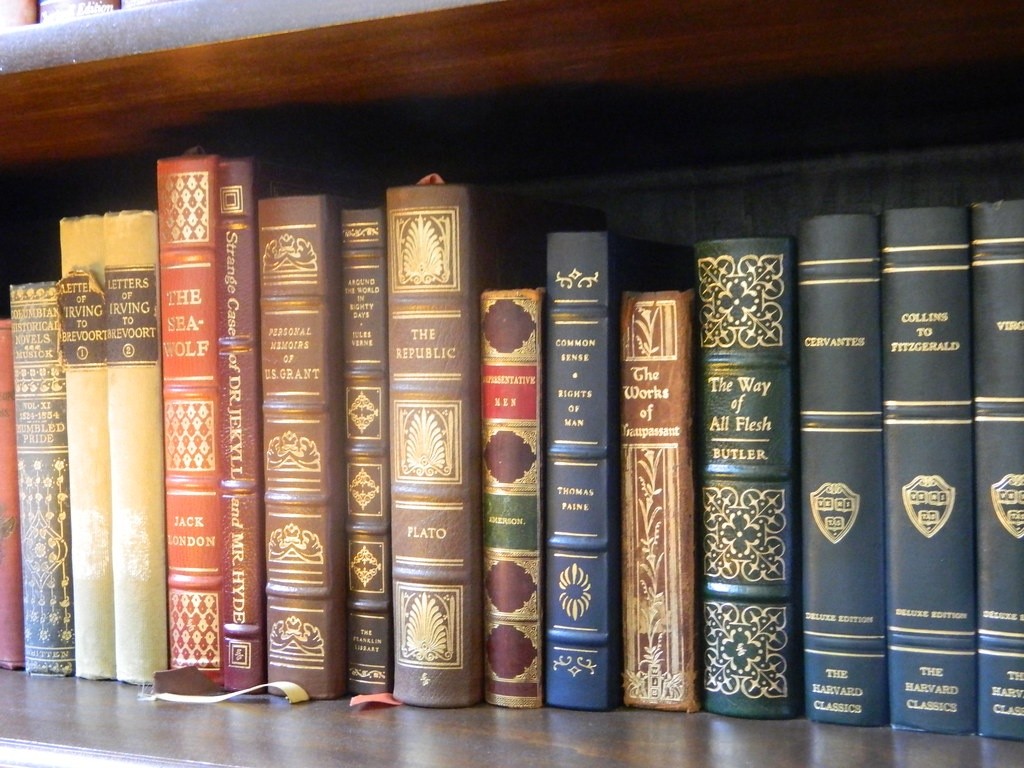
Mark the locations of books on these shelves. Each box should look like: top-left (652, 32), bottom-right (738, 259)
top-left (8, 280), bottom-right (77, 680)
top-left (536, 226), bottom-right (623, 711)
top-left (478, 281), bottom-right (551, 714)
top-left (797, 210), bottom-right (890, 722)
top-left (59, 214), bottom-right (120, 685)
top-left (152, 151), bottom-right (227, 695)
top-left (618, 278), bottom-right (704, 711)
top-left (880, 203), bottom-right (975, 733)
top-left (0, 316), bottom-right (23, 670)
top-left (386, 173), bottom-right (483, 709)
top-left (692, 232), bottom-right (800, 718)
top-left (974, 193), bottom-right (1024, 746)
top-left (252, 188), bottom-right (350, 699)
top-left (101, 210), bottom-right (174, 694)
top-left (341, 204), bottom-right (392, 699)
top-left (220, 155), bottom-right (264, 703)
